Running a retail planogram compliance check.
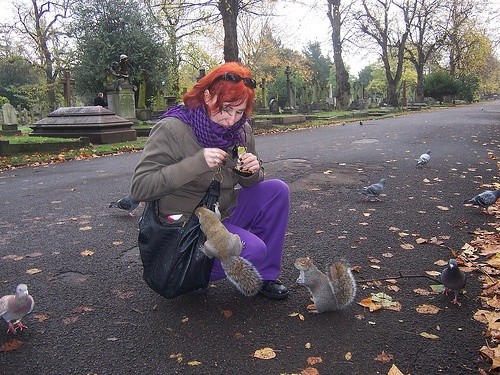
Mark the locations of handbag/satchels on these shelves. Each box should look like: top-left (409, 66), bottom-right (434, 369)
top-left (137, 179), bottom-right (221, 300)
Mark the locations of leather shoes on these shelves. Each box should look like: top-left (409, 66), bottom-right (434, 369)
top-left (261, 280), bottom-right (289, 299)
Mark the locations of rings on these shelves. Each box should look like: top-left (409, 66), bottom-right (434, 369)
top-left (216, 153), bottom-right (219, 158)
top-left (212, 158), bottom-right (216, 162)
top-left (212, 148), bottom-right (216, 153)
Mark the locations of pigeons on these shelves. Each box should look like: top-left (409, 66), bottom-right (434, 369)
top-left (361, 179), bottom-right (387, 202)
top-left (0, 283), bottom-right (34, 333)
top-left (416, 149), bottom-right (431, 167)
top-left (441, 259), bottom-right (467, 307)
top-left (109, 194), bottom-right (140, 218)
top-left (463, 188), bottom-right (500, 215)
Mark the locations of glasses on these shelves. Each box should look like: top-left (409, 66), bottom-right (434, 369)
top-left (216, 72), bottom-right (257, 89)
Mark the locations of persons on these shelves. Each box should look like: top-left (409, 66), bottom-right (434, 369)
top-left (110, 55), bottom-right (135, 92)
top-left (93, 92), bottom-right (104, 107)
top-left (131, 61), bottom-right (290, 300)
